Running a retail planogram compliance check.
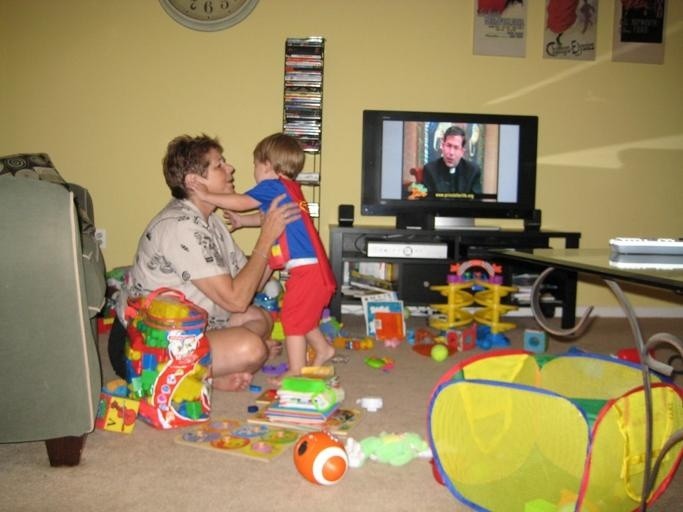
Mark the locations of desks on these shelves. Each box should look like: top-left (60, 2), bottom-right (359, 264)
top-left (492, 245), bottom-right (682, 347)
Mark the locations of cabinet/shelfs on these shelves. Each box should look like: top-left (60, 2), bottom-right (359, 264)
top-left (321, 224), bottom-right (584, 341)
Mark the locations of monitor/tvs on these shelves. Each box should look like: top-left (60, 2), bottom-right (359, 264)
top-left (361, 109), bottom-right (538, 231)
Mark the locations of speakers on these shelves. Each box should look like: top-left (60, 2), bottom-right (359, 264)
top-left (524, 209), bottom-right (541, 232)
top-left (338, 205), bottom-right (354, 227)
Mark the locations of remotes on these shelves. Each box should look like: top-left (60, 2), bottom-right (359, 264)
top-left (609, 253), bottom-right (683, 271)
top-left (608, 237), bottom-right (683, 255)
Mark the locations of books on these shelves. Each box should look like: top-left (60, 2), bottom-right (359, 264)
top-left (174, 376), bottom-right (363, 460)
top-left (283, 36), bottom-right (324, 154)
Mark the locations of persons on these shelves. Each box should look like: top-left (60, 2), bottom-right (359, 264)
top-left (423, 126), bottom-right (482, 202)
top-left (117, 132), bottom-right (302, 392)
top-left (185, 133), bottom-right (337, 384)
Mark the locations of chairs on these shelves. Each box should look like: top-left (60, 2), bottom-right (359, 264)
top-left (0, 173), bottom-right (109, 469)
